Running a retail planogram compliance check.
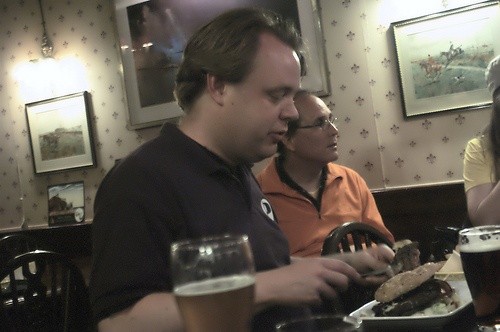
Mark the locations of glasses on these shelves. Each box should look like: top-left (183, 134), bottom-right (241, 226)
top-left (290, 114), bottom-right (336, 131)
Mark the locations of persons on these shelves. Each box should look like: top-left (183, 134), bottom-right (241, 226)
top-left (255, 89), bottom-right (394, 258)
top-left (88, 5), bottom-right (396, 332)
top-left (462, 54), bottom-right (500, 227)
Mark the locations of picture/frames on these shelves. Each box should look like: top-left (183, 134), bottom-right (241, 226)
top-left (25, 90), bottom-right (97, 178)
top-left (109, 0), bottom-right (332, 130)
top-left (389, 0), bottom-right (500, 121)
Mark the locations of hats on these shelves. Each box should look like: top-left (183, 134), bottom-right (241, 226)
top-left (485, 56), bottom-right (500, 96)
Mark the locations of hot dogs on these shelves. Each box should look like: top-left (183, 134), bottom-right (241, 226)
top-left (372, 262), bottom-right (454, 317)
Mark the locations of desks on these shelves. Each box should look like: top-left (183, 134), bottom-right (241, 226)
top-left (0, 182), bottom-right (473, 275)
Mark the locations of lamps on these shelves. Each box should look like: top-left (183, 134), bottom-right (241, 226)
top-left (29, 0), bottom-right (55, 60)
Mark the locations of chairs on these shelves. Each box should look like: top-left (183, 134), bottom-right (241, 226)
top-left (322, 223), bottom-right (393, 257)
top-left (0, 233), bottom-right (95, 332)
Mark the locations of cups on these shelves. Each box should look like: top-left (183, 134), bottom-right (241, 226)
top-left (457, 226), bottom-right (500, 331)
top-left (170, 235), bottom-right (257, 331)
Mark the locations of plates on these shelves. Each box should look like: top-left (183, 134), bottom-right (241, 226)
top-left (348, 271), bottom-right (472, 331)
